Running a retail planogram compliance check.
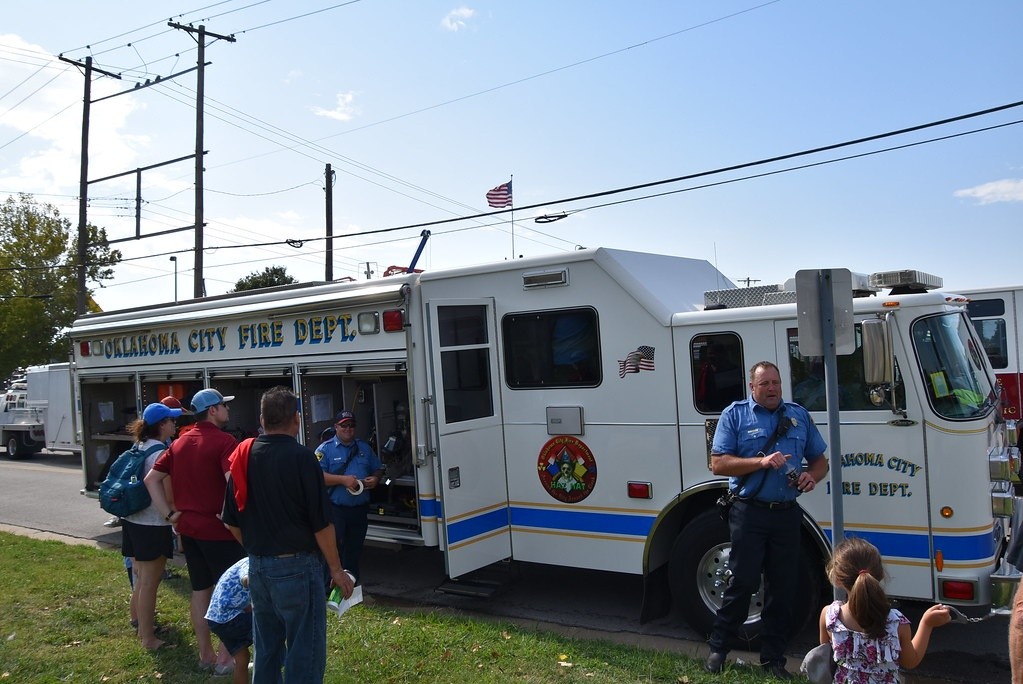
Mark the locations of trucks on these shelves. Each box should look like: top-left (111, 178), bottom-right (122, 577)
top-left (0, 361), bottom-right (81, 461)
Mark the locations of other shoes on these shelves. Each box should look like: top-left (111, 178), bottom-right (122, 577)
top-left (214, 662), bottom-right (254, 678)
top-left (199, 661), bottom-right (216, 669)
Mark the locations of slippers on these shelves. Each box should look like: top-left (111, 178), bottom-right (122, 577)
top-left (130, 620), bottom-right (161, 634)
top-left (157, 642), bottom-right (179, 651)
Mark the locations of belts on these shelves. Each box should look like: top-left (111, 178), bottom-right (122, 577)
top-left (735, 498), bottom-right (795, 510)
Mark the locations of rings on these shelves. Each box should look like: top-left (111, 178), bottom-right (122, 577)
top-left (809, 481), bottom-right (814, 483)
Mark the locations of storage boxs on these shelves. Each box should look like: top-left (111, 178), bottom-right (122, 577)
top-left (158, 382), bottom-right (191, 406)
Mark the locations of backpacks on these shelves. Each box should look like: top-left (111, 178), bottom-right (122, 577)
top-left (98, 443), bottom-right (166, 517)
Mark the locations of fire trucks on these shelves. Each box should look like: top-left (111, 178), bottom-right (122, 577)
top-left (51, 250), bottom-right (1023, 652)
top-left (943, 286), bottom-right (1023, 421)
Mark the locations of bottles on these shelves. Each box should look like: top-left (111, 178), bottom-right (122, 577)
top-left (783, 461), bottom-right (803, 493)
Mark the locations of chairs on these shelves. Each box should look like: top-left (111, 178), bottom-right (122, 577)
top-left (704, 343), bottom-right (742, 411)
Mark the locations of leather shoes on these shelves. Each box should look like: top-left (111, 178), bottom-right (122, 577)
top-left (704, 647), bottom-right (726, 674)
top-left (765, 666), bottom-right (794, 681)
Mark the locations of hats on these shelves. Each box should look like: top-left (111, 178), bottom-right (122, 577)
top-left (161, 395), bottom-right (188, 413)
top-left (336, 410), bottom-right (355, 425)
top-left (142, 403), bottom-right (182, 424)
top-left (190, 389), bottom-right (235, 415)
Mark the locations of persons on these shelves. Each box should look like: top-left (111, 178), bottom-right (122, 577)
top-left (314, 411), bottom-right (385, 588)
top-left (820, 537), bottom-right (952, 684)
top-left (706, 361), bottom-right (828, 682)
top-left (1007, 521), bottom-right (1023, 684)
top-left (203, 385), bottom-right (354, 684)
top-left (103, 396), bottom-right (196, 651)
top-left (143, 388), bottom-right (252, 678)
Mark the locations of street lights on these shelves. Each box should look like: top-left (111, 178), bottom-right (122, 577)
top-left (169, 256), bottom-right (177, 303)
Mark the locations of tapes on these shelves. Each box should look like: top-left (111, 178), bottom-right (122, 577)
top-left (348, 480), bottom-right (363, 496)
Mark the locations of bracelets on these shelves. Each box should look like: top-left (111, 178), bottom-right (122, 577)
top-left (166, 511), bottom-right (175, 521)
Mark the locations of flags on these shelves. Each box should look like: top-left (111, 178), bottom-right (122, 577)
top-left (486, 180), bottom-right (512, 208)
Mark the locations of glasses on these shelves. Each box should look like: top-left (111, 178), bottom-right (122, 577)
top-left (340, 422), bottom-right (355, 428)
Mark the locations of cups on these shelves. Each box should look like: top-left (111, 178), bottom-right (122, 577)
top-left (326, 567), bottom-right (357, 610)
